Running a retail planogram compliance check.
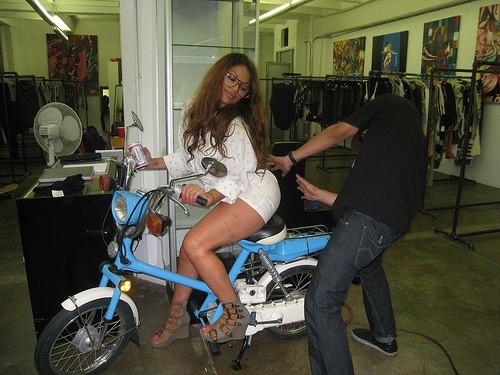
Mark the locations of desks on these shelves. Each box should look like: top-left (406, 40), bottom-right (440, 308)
top-left (15, 155), bottom-right (120, 341)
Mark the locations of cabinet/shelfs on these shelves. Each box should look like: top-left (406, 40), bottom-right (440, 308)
top-left (165, 0), bottom-right (260, 276)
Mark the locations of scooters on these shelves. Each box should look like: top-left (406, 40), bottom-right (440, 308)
top-left (33, 108), bottom-right (333, 375)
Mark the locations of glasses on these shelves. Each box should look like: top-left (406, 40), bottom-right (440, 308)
top-left (224, 70), bottom-right (252, 98)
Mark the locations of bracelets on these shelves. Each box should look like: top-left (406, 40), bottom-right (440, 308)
top-left (205, 192), bottom-right (214, 206)
top-left (288, 151), bottom-right (299, 165)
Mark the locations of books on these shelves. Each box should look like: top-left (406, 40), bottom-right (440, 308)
top-left (39, 166), bottom-right (95, 183)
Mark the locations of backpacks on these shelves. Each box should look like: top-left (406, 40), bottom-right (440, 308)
top-left (82, 126), bottom-right (108, 153)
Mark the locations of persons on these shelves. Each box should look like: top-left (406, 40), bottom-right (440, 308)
top-left (124, 53), bottom-right (281, 347)
top-left (267, 94), bottom-right (429, 375)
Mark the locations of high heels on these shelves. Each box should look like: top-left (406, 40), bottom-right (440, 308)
top-left (151, 300), bottom-right (190, 347)
top-left (198, 298), bottom-right (251, 342)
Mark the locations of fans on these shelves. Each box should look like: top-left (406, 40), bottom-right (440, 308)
top-left (33, 102), bottom-right (82, 166)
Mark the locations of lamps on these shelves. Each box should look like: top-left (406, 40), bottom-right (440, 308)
top-left (248, 0), bottom-right (313, 25)
top-left (25, 0), bottom-right (73, 40)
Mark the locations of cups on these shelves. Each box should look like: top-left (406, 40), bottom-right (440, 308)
top-left (118, 127), bottom-right (125, 138)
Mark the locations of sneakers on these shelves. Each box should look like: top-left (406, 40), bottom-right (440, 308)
top-left (351, 328), bottom-right (398, 356)
